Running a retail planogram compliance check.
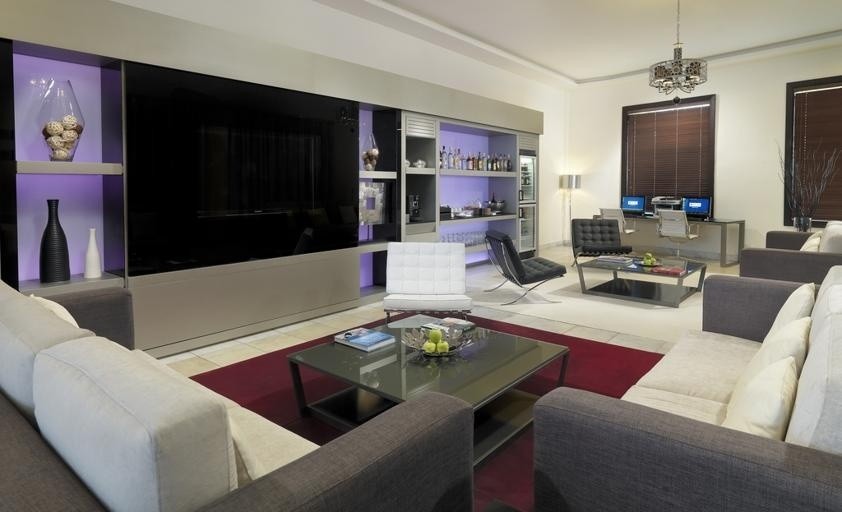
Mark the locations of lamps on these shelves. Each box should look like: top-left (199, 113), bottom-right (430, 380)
top-left (648, 2), bottom-right (709, 96)
top-left (559, 173), bottom-right (582, 251)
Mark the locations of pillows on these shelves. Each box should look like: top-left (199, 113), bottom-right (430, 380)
top-left (755, 316), bottom-right (810, 375)
top-left (723, 355), bottom-right (797, 440)
top-left (763, 281), bottom-right (815, 323)
top-left (800, 230), bottom-right (823, 252)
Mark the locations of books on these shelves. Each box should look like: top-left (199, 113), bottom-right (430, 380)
top-left (334, 328), bottom-right (395, 353)
top-left (652, 265), bottom-right (685, 276)
top-left (595, 256), bottom-right (632, 265)
top-left (421, 316), bottom-right (476, 335)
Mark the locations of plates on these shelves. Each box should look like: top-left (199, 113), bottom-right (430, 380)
top-left (631, 256), bottom-right (664, 268)
top-left (399, 325), bottom-right (471, 358)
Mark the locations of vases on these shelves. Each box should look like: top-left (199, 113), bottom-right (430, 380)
top-left (361, 127), bottom-right (381, 172)
top-left (39, 196), bottom-right (72, 285)
top-left (84, 229), bottom-right (104, 281)
top-left (34, 78), bottom-right (85, 163)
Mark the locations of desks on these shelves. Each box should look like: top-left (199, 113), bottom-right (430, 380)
top-left (596, 213), bottom-right (746, 269)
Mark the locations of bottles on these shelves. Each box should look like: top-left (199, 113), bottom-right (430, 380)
top-left (496, 153), bottom-right (500, 171)
top-left (461, 154), bottom-right (467, 170)
top-left (440, 149), bottom-right (442, 169)
top-left (492, 153), bottom-right (496, 171)
top-left (477, 151), bottom-right (482, 170)
top-left (507, 153), bottom-right (512, 172)
top-left (467, 152), bottom-right (473, 170)
top-left (499, 153), bottom-right (503, 170)
top-left (487, 154), bottom-right (491, 171)
top-left (442, 146), bottom-right (448, 169)
top-left (453, 149), bottom-right (460, 169)
top-left (448, 145), bottom-right (453, 169)
top-left (457, 148), bottom-right (462, 157)
top-left (482, 152), bottom-right (487, 171)
top-left (503, 152), bottom-right (507, 171)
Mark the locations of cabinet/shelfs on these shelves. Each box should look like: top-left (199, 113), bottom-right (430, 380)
top-left (402, 109), bottom-right (540, 269)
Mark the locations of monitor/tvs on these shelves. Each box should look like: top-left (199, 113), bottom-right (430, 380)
top-left (680, 195), bottom-right (712, 221)
top-left (620, 195), bottom-right (646, 217)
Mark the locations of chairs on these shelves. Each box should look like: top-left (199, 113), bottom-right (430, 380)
top-left (570, 218), bottom-right (635, 280)
top-left (654, 209), bottom-right (701, 263)
top-left (485, 230), bottom-right (567, 304)
top-left (598, 209), bottom-right (637, 252)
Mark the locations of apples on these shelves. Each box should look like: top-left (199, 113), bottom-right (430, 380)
top-left (428, 329), bottom-right (441, 343)
top-left (437, 341), bottom-right (449, 353)
top-left (643, 260), bottom-right (650, 264)
top-left (423, 341), bottom-right (436, 354)
top-left (650, 258), bottom-right (656, 265)
top-left (644, 252), bottom-right (652, 259)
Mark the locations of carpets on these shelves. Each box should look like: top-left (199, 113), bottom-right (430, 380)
top-left (189, 311), bottom-right (664, 512)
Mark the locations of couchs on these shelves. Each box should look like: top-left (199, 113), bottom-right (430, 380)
top-left (0, 276), bottom-right (477, 511)
top-left (383, 241), bottom-right (474, 325)
top-left (532, 263), bottom-right (842, 510)
top-left (739, 220), bottom-right (842, 286)
